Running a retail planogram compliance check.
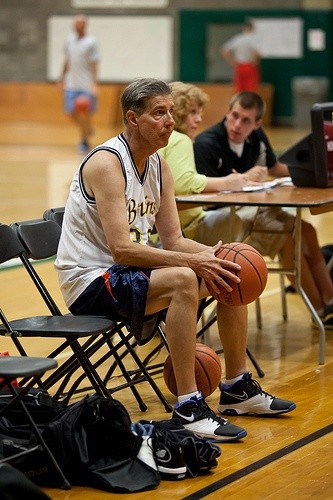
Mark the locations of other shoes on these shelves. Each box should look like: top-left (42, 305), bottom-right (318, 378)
top-left (81, 141), bottom-right (88, 151)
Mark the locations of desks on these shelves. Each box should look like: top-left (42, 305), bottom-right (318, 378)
top-left (176, 186), bottom-right (333, 364)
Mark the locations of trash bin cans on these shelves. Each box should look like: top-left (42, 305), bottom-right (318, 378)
top-left (290, 75), bottom-right (328, 128)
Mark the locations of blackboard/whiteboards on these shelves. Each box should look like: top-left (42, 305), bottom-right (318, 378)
top-left (248, 15), bottom-right (303, 59)
top-left (46, 15), bottom-right (176, 84)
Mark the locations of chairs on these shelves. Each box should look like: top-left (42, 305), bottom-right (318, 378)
top-left (0, 206), bottom-right (265, 492)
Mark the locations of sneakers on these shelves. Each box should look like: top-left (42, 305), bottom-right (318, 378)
top-left (130, 428), bottom-right (186, 480)
top-left (172, 396), bottom-right (247, 443)
top-left (218, 372), bottom-right (296, 416)
top-left (311, 303), bottom-right (333, 329)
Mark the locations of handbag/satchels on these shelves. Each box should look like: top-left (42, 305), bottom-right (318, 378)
top-left (0, 388), bottom-right (161, 500)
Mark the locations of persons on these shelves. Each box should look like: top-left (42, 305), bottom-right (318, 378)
top-left (54, 79), bottom-right (297, 443)
top-left (53, 14), bottom-right (333, 334)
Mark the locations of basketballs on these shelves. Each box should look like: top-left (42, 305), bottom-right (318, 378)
top-left (204, 241), bottom-right (269, 305)
top-left (164, 342), bottom-right (223, 399)
